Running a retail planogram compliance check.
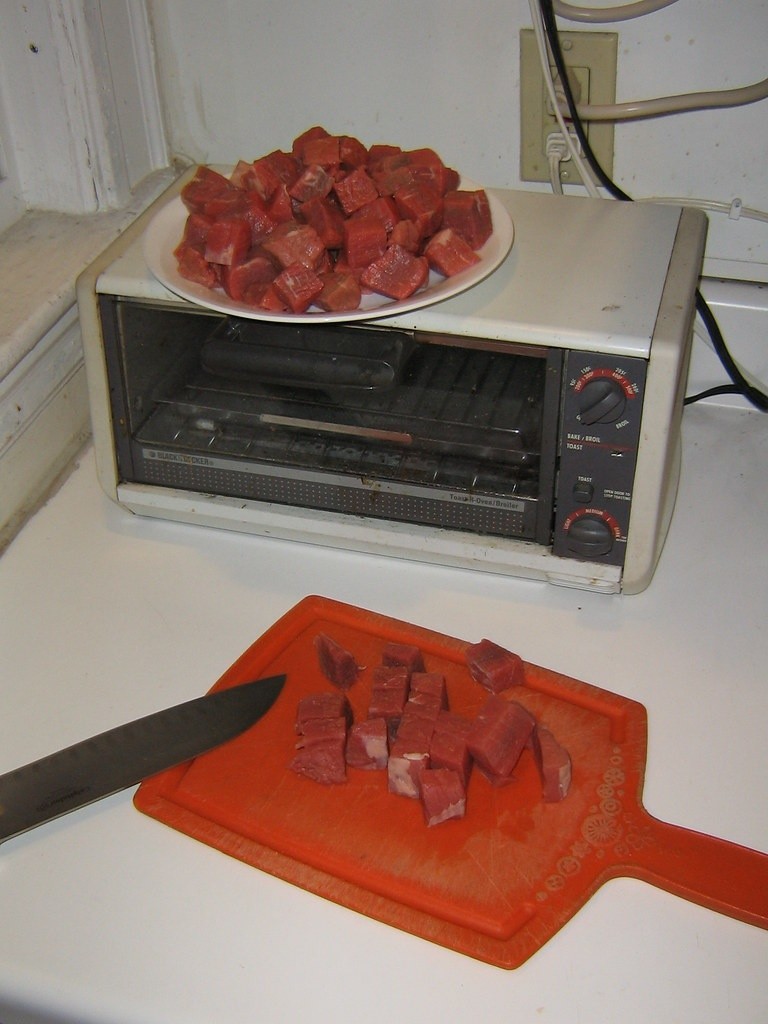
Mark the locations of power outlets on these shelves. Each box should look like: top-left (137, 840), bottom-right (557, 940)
top-left (519, 28), bottom-right (618, 186)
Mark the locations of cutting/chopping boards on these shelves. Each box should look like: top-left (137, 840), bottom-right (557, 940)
top-left (133, 594), bottom-right (768, 969)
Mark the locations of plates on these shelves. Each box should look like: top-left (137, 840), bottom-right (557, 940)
top-left (144, 175), bottom-right (514, 324)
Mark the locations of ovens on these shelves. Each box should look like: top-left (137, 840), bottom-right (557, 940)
top-left (76, 164), bottom-right (707, 595)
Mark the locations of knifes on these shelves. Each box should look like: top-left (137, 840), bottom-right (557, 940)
top-left (1, 674), bottom-right (286, 844)
